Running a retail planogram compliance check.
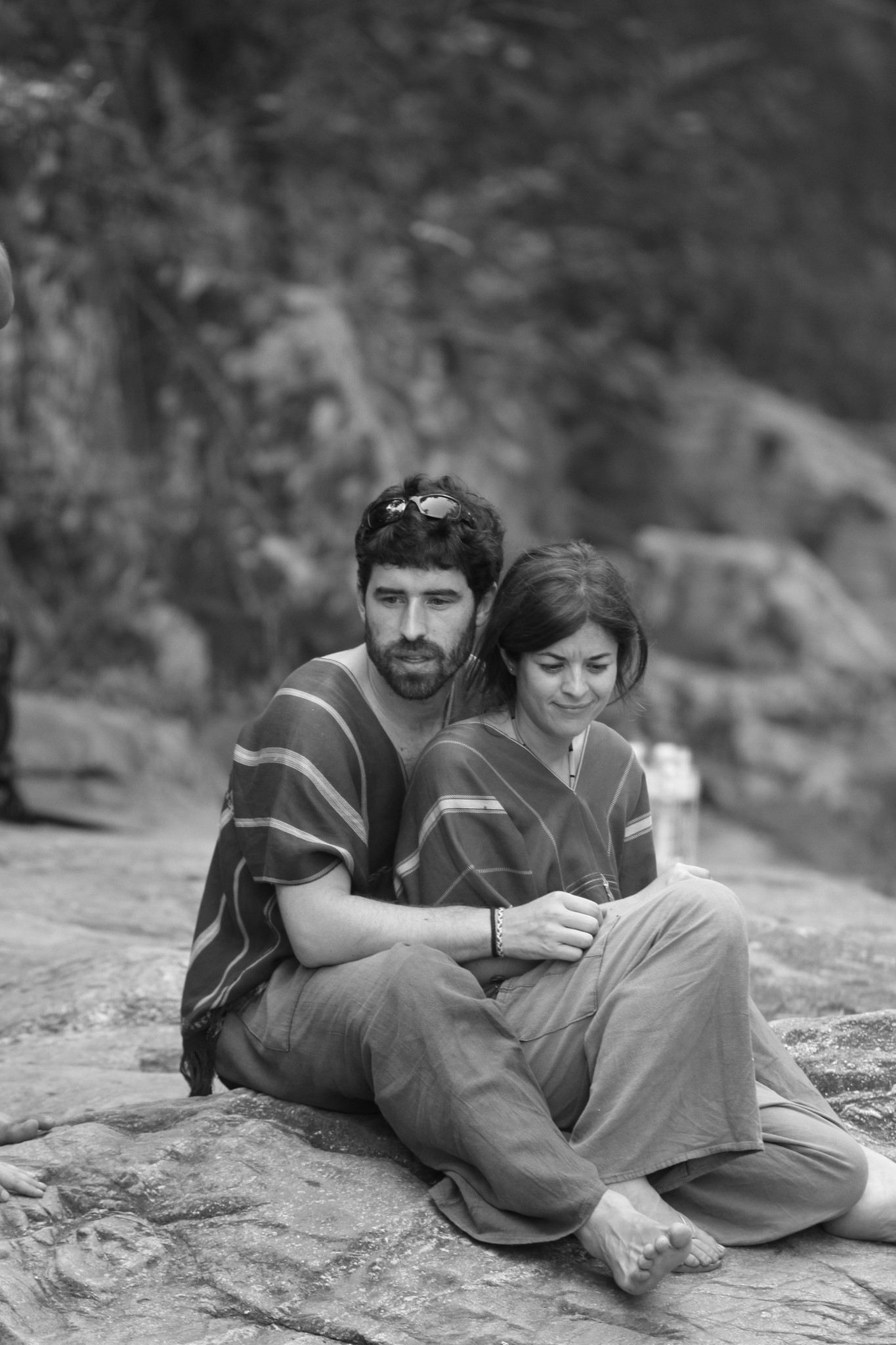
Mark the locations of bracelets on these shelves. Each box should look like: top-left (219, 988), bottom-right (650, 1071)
top-left (490, 907), bottom-right (498, 958)
top-left (496, 907), bottom-right (504, 958)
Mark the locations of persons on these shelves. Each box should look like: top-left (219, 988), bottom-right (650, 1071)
top-left (179, 474), bottom-right (896, 1298)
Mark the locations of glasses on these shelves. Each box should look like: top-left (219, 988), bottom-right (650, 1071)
top-left (365, 493), bottom-right (477, 545)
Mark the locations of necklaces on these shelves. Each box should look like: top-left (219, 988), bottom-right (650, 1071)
top-left (509, 700), bottom-right (576, 789)
